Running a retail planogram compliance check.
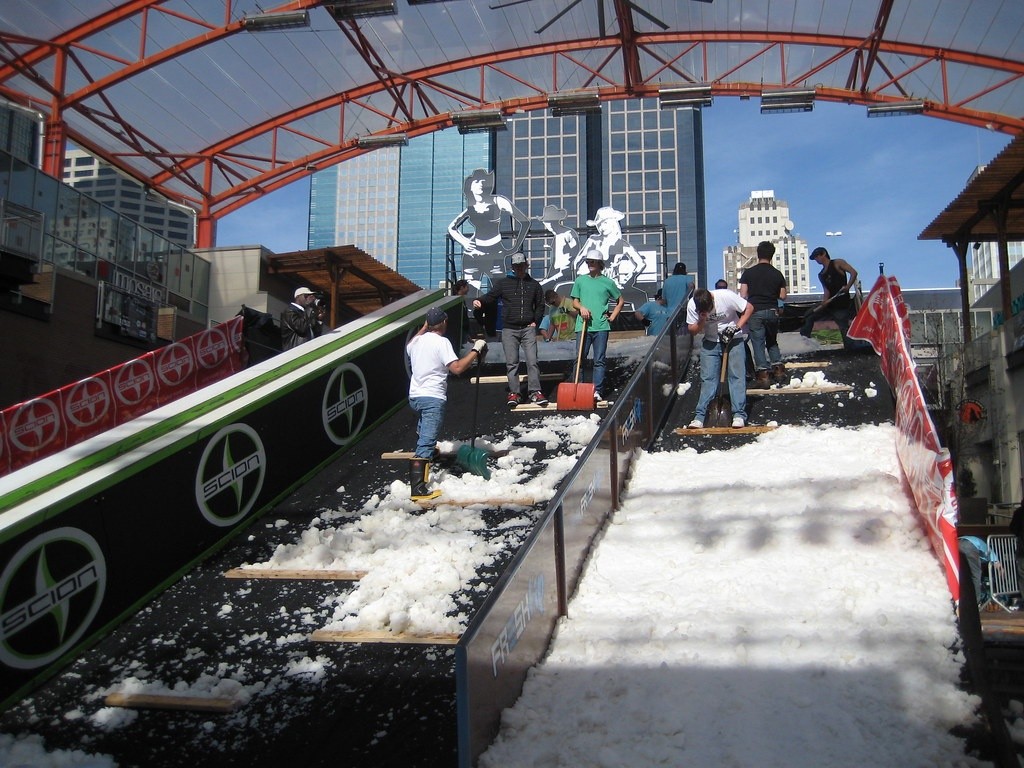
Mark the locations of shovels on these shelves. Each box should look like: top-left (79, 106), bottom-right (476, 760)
top-left (454, 341), bottom-right (492, 480)
top-left (778, 287), bottom-right (846, 332)
top-left (701, 331), bottom-right (739, 428)
top-left (555, 309), bottom-right (598, 413)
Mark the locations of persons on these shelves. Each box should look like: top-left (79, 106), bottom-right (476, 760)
top-left (958, 536), bottom-right (1004, 611)
top-left (539, 315), bottom-right (558, 342)
top-left (657, 262), bottom-right (695, 321)
top-left (635, 289), bottom-right (668, 336)
top-left (406, 307), bottom-right (486, 502)
top-left (1008, 498), bottom-right (1024, 609)
top-left (453, 279), bottom-right (470, 296)
top-left (686, 289), bottom-right (754, 429)
top-left (715, 279), bottom-right (728, 289)
top-left (739, 241), bottom-right (787, 389)
top-left (799, 247), bottom-right (858, 350)
top-left (570, 249), bottom-right (624, 402)
top-left (280, 287), bottom-right (326, 352)
top-left (472, 252), bottom-right (548, 406)
top-left (544, 289), bottom-right (579, 343)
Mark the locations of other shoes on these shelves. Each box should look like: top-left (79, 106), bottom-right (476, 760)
top-left (688, 420), bottom-right (704, 429)
top-left (594, 392), bottom-right (602, 401)
top-left (732, 418), bottom-right (745, 427)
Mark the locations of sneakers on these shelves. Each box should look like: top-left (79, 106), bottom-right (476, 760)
top-left (528, 391), bottom-right (548, 405)
top-left (507, 393), bottom-right (521, 406)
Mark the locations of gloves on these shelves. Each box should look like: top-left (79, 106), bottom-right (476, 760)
top-left (722, 322), bottom-right (739, 336)
top-left (471, 340), bottom-right (486, 354)
top-left (642, 318), bottom-right (652, 326)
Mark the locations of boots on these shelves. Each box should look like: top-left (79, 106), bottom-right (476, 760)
top-left (770, 366), bottom-right (785, 384)
top-left (409, 458), bottom-right (442, 501)
top-left (747, 369), bottom-right (770, 389)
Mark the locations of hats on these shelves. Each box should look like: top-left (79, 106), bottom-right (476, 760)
top-left (294, 287), bottom-right (317, 298)
top-left (675, 263), bottom-right (685, 268)
top-left (810, 247), bottom-right (825, 260)
top-left (586, 250), bottom-right (603, 261)
top-left (427, 307), bottom-right (448, 325)
top-left (511, 253), bottom-right (526, 265)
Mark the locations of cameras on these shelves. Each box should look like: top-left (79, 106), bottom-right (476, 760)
top-left (315, 299), bottom-right (323, 307)
